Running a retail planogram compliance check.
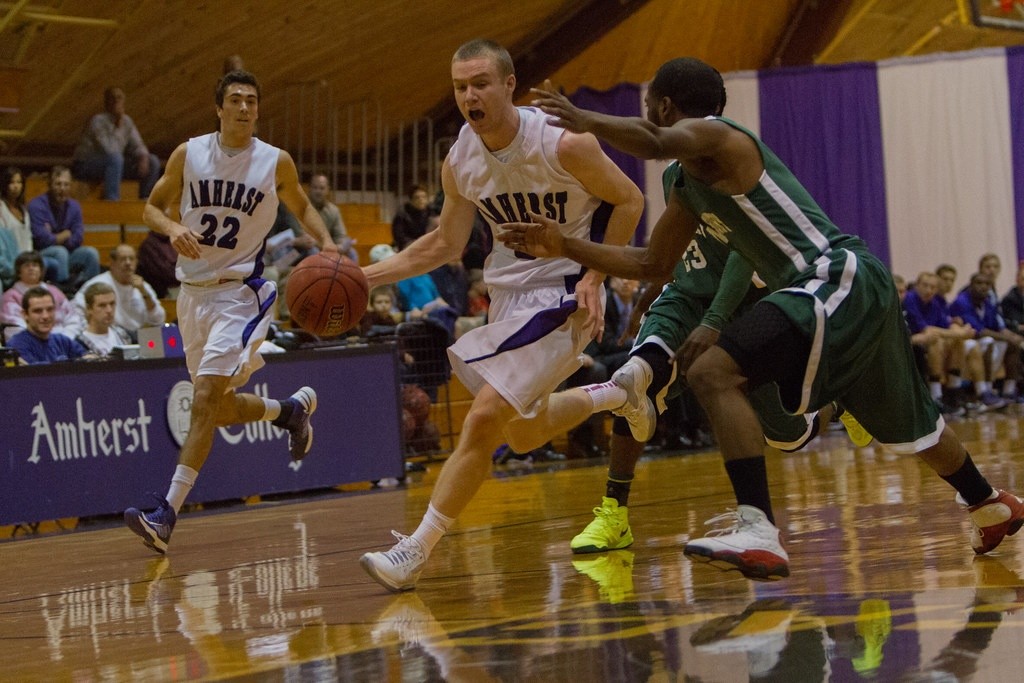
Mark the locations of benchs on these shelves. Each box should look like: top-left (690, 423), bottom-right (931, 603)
top-left (26, 180), bottom-right (612, 464)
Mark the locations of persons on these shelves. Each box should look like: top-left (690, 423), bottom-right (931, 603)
top-left (72, 243), bottom-right (164, 330)
top-left (0, 251), bottom-right (82, 345)
top-left (262, 173), bottom-right (361, 351)
top-left (369, 183), bottom-right (493, 320)
top-left (124, 70), bottom-right (340, 554)
top-left (71, 86), bottom-right (160, 200)
top-left (893, 255), bottom-right (1024, 418)
top-left (404, 384), bottom-right (440, 453)
top-left (496, 57), bottom-right (1024, 583)
top-left (134, 230), bottom-right (181, 299)
top-left (361, 41), bottom-right (658, 593)
top-left (492, 276), bottom-right (713, 466)
top-left (0, 167), bottom-right (76, 296)
top-left (7, 286), bottom-right (96, 364)
top-left (76, 282), bottom-right (132, 359)
top-left (28, 165), bottom-right (100, 291)
top-left (369, 286), bottom-right (414, 384)
top-left (570, 64), bottom-right (873, 551)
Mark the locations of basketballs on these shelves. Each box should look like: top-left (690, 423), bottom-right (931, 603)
top-left (401, 386), bottom-right (442, 453)
top-left (285, 251), bottom-right (371, 339)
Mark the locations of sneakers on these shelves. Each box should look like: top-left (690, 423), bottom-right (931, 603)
top-left (955, 486), bottom-right (1024, 554)
top-left (609, 355), bottom-right (659, 443)
top-left (684, 504), bottom-right (790, 583)
top-left (358, 529), bottom-right (428, 592)
top-left (935, 386), bottom-right (1024, 418)
top-left (570, 495), bottom-right (634, 553)
top-left (271, 386), bottom-right (318, 460)
top-left (123, 489), bottom-right (177, 555)
top-left (838, 410), bottom-right (874, 447)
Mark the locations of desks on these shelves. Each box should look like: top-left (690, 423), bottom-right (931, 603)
top-left (0, 341), bottom-right (408, 528)
